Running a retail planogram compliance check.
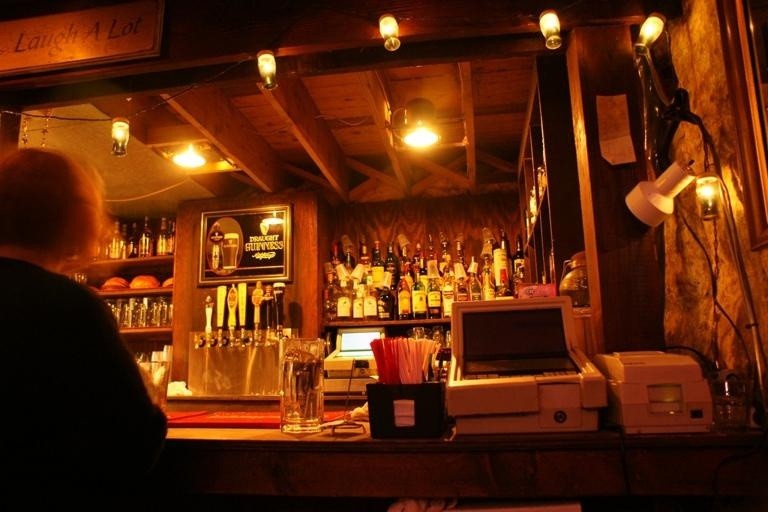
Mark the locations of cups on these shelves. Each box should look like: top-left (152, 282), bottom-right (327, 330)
top-left (136, 360), bottom-right (172, 414)
top-left (222, 233), bottom-right (239, 268)
top-left (712, 376), bottom-right (751, 431)
top-left (278, 338), bottom-right (325, 433)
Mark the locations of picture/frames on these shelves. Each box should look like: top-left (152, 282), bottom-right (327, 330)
top-left (198, 202), bottom-right (294, 283)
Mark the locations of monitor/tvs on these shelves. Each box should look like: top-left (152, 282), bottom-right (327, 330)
top-left (462, 306), bottom-right (570, 361)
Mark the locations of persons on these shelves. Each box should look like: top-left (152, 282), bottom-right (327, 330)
top-left (0, 148), bottom-right (167, 512)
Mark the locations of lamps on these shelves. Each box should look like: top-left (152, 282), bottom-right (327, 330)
top-left (694, 172), bottom-right (720, 220)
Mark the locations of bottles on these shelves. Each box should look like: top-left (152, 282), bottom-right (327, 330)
top-left (109, 215), bottom-right (175, 260)
top-left (559, 250), bottom-right (590, 307)
top-left (323, 234), bottom-right (527, 322)
top-left (112, 296), bottom-right (173, 328)
top-left (412, 326), bottom-right (451, 383)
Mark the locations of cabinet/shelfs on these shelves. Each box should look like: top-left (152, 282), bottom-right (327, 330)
top-left (517, 53), bottom-right (583, 289)
top-left (79, 255), bottom-right (175, 340)
